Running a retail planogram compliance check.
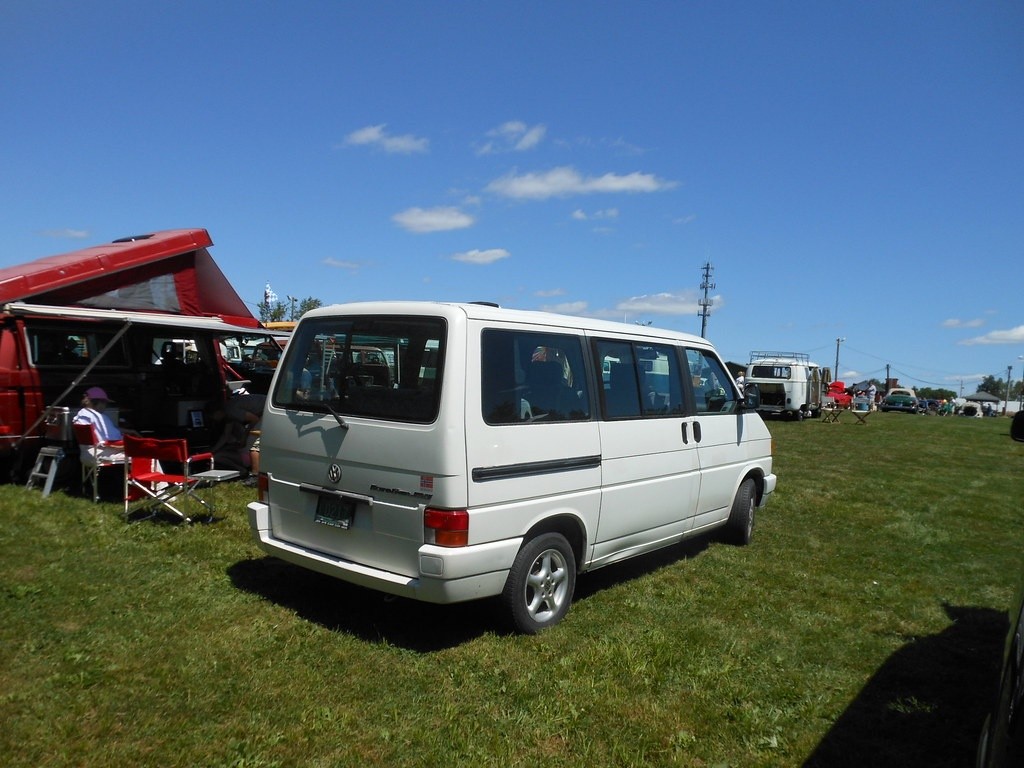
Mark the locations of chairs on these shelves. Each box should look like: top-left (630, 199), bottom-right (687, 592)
top-left (532, 359), bottom-right (579, 420)
top-left (73, 422), bottom-right (153, 505)
top-left (604, 363), bottom-right (651, 417)
top-left (123, 433), bottom-right (240, 530)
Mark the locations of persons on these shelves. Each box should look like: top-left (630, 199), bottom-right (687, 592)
top-left (865, 380), bottom-right (876, 412)
top-left (922, 397), bottom-right (957, 417)
top-left (202, 393), bottom-right (268, 487)
top-left (525, 345), bottom-right (573, 410)
top-left (72, 387), bottom-right (177, 503)
top-left (296, 353), bottom-right (312, 403)
top-left (703, 369), bottom-right (745, 400)
top-left (986, 404), bottom-right (992, 416)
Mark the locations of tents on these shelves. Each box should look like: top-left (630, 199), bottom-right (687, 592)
top-left (966, 390), bottom-right (1001, 417)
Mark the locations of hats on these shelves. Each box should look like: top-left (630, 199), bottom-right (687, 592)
top-left (84, 387), bottom-right (114, 402)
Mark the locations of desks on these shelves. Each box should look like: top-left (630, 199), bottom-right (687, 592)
top-left (821, 408), bottom-right (843, 423)
top-left (852, 409), bottom-right (871, 424)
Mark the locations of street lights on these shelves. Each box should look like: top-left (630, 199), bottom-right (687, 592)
top-left (697, 262), bottom-right (716, 378)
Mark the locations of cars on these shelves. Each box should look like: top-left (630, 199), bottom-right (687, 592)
top-left (880, 389), bottom-right (919, 413)
top-left (827, 388), bottom-right (852, 410)
top-left (958, 402), bottom-right (984, 418)
top-left (918, 399), bottom-right (939, 414)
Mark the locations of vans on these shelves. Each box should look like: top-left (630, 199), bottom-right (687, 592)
top-left (0, 302), bottom-right (285, 494)
top-left (250, 339), bottom-right (391, 394)
top-left (742, 350), bottom-right (824, 421)
top-left (246, 299), bottom-right (777, 633)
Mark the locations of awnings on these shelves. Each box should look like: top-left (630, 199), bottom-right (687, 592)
top-left (3, 301), bottom-right (295, 337)
